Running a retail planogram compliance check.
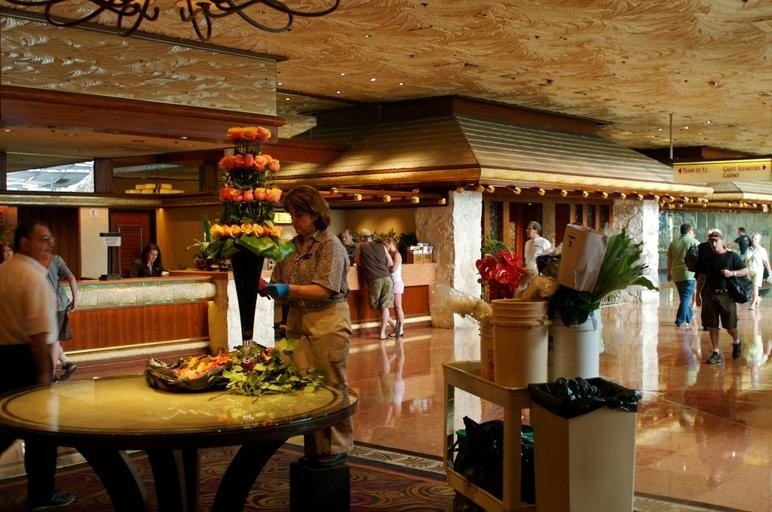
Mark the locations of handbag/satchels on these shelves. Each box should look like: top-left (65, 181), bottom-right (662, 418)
top-left (685, 242), bottom-right (699, 272)
top-left (723, 250), bottom-right (753, 304)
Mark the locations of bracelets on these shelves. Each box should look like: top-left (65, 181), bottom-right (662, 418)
top-left (295, 285), bottom-right (301, 300)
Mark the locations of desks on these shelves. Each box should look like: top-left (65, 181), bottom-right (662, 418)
top-left (1, 373), bottom-right (359, 511)
top-left (441, 359), bottom-right (532, 511)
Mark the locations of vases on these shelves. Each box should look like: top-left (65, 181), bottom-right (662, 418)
top-left (229, 245), bottom-right (269, 340)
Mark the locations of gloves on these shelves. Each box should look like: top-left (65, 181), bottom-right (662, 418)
top-left (266, 283), bottom-right (289, 299)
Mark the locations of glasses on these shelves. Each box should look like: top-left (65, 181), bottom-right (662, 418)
top-left (28, 235), bottom-right (55, 243)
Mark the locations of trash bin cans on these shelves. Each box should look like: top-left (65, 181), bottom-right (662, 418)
top-left (528, 377), bottom-right (642, 512)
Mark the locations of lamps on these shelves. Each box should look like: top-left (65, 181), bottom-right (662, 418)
top-left (124, 183), bottom-right (183, 196)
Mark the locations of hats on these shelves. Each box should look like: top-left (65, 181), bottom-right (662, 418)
top-left (709, 232), bottom-right (723, 241)
top-left (358, 229), bottom-right (371, 236)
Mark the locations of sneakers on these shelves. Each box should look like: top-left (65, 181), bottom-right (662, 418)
top-left (58, 362), bottom-right (76, 381)
top-left (749, 304), bottom-right (756, 310)
top-left (30, 491), bottom-right (76, 511)
top-left (756, 296), bottom-right (762, 307)
top-left (733, 338), bottom-right (741, 359)
top-left (395, 320), bottom-right (401, 339)
top-left (707, 352), bottom-right (722, 364)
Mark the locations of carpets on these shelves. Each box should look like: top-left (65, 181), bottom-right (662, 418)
top-left (1, 434), bottom-right (746, 512)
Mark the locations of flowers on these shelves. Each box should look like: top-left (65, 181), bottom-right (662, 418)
top-left (184, 124), bottom-right (300, 262)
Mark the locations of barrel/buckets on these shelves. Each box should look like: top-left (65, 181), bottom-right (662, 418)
top-left (478, 319), bottom-right (495, 382)
top-left (548, 309), bottom-right (603, 380)
top-left (490, 299), bottom-right (554, 387)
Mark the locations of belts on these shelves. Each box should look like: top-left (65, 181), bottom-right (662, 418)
top-left (289, 300), bottom-right (320, 308)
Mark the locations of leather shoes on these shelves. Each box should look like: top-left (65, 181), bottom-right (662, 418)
top-left (318, 452), bottom-right (348, 467)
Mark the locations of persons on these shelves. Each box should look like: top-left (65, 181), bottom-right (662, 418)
top-left (693, 364), bottom-right (752, 490)
top-left (384, 235), bottom-right (404, 338)
top-left (341, 226), bottom-right (357, 266)
top-left (0, 240), bottom-right (14, 263)
top-left (41, 254), bottom-right (78, 384)
top-left (0, 220), bottom-right (78, 511)
top-left (666, 223), bottom-right (772, 365)
top-left (377, 337), bottom-right (405, 428)
top-left (257, 185), bottom-right (355, 468)
top-left (354, 228), bottom-right (395, 340)
top-left (524, 220), bottom-right (551, 274)
top-left (129, 243), bottom-right (167, 276)
